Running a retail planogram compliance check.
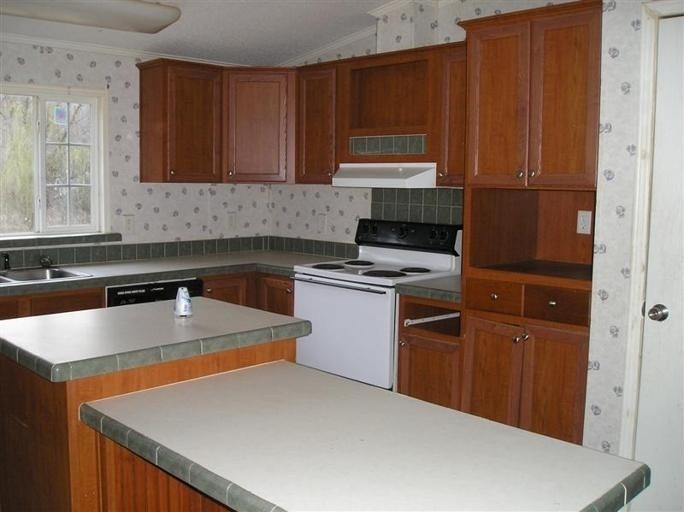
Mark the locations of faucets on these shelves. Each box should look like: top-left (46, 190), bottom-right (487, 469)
top-left (0, 252), bottom-right (11, 270)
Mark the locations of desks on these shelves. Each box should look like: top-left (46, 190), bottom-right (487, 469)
top-left (1, 288), bottom-right (314, 512)
top-left (76, 358), bottom-right (652, 512)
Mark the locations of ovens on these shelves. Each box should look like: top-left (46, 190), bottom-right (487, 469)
top-left (289, 276), bottom-right (396, 392)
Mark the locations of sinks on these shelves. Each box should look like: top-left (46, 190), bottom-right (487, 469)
top-left (0, 277), bottom-right (11, 284)
top-left (0, 268), bottom-right (94, 281)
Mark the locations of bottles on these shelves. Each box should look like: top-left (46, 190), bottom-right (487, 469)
top-left (174, 286), bottom-right (193, 319)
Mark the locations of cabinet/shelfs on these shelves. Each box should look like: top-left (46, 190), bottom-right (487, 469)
top-left (223, 64), bottom-right (296, 185)
top-left (339, 39), bottom-right (464, 188)
top-left (460, 264), bottom-right (593, 446)
top-left (136, 58), bottom-right (221, 184)
top-left (0, 283), bottom-right (107, 319)
top-left (296, 62), bottom-right (337, 185)
top-left (249, 274), bottom-right (293, 316)
top-left (201, 272), bottom-right (248, 308)
top-left (458, 1), bottom-right (603, 189)
top-left (396, 284), bottom-right (463, 411)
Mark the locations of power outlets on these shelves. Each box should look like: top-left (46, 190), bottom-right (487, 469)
top-left (576, 210), bottom-right (592, 234)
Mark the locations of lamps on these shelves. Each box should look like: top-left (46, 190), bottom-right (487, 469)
top-left (0, 2), bottom-right (183, 36)
